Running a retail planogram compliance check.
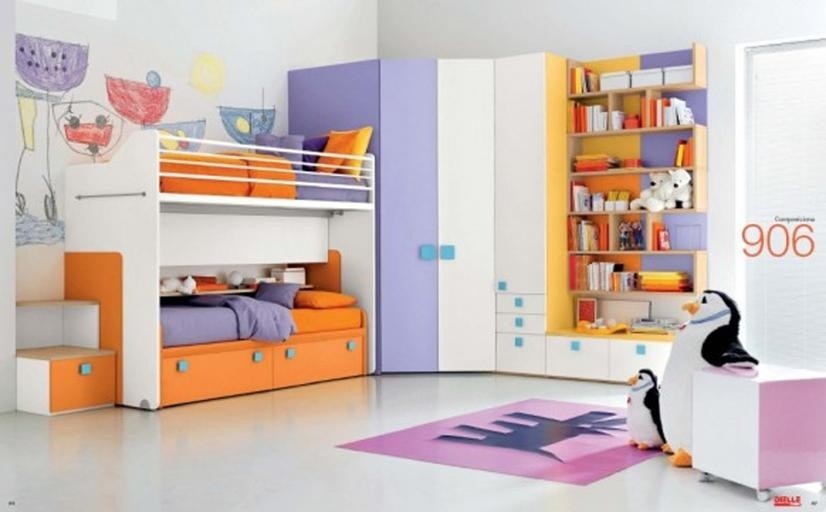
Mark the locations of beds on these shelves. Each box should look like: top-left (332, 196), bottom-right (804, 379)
top-left (65, 127), bottom-right (377, 410)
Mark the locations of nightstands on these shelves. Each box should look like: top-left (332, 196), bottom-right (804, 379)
top-left (16, 345), bottom-right (117, 416)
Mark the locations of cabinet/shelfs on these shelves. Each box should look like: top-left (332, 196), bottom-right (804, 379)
top-left (692, 366), bottom-right (826, 502)
top-left (546, 340), bottom-right (672, 387)
top-left (546, 43), bottom-right (709, 341)
top-left (494, 52), bottom-right (546, 376)
top-left (288, 60), bottom-right (495, 375)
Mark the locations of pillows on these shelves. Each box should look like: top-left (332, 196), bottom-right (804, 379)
top-left (251, 283), bottom-right (301, 309)
top-left (315, 130), bottom-right (362, 177)
top-left (302, 136), bottom-right (328, 171)
top-left (255, 130), bottom-right (308, 171)
top-left (294, 290), bottom-right (357, 310)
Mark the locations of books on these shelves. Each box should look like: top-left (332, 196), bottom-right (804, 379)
top-left (567, 63), bottom-right (693, 292)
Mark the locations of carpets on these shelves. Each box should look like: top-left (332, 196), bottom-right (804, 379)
top-left (334, 400), bottom-right (665, 486)
